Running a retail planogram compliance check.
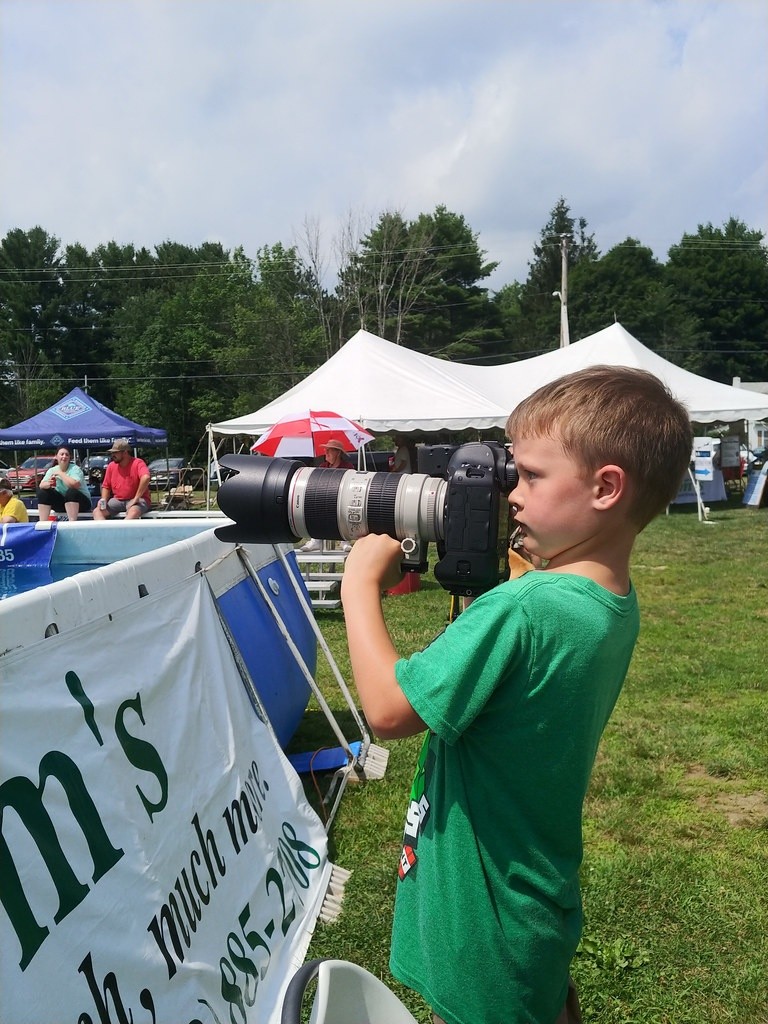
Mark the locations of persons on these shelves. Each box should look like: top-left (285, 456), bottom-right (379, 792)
top-left (0, 478), bottom-right (29, 523)
top-left (37, 446), bottom-right (92, 521)
top-left (319, 440), bottom-right (353, 469)
top-left (342, 364), bottom-right (689, 1024)
top-left (93, 439), bottom-right (151, 520)
top-left (389, 436), bottom-right (410, 473)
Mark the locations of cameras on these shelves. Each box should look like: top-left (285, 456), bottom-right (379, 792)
top-left (214, 439), bottom-right (518, 599)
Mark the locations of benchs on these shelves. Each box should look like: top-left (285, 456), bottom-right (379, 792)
top-left (27, 507), bottom-right (351, 610)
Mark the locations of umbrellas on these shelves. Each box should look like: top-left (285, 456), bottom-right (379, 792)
top-left (249, 409), bottom-right (375, 466)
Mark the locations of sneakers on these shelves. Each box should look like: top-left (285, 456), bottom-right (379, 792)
top-left (300, 539), bottom-right (319, 552)
top-left (340, 541), bottom-right (351, 552)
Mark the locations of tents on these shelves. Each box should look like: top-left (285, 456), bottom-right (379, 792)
top-left (0, 387), bottom-right (170, 505)
top-left (207, 322), bottom-right (768, 521)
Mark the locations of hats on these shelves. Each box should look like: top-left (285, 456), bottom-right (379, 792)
top-left (0, 478), bottom-right (12, 490)
top-left (320, 439), bottom-right (350, 458)
top-left (107, 439), bottom-right (128, 452)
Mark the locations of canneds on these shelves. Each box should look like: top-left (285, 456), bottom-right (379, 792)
top-left (50, 476), bottom-right (57, 488)
top-left (100, 499), bottom-right (106, 510)
